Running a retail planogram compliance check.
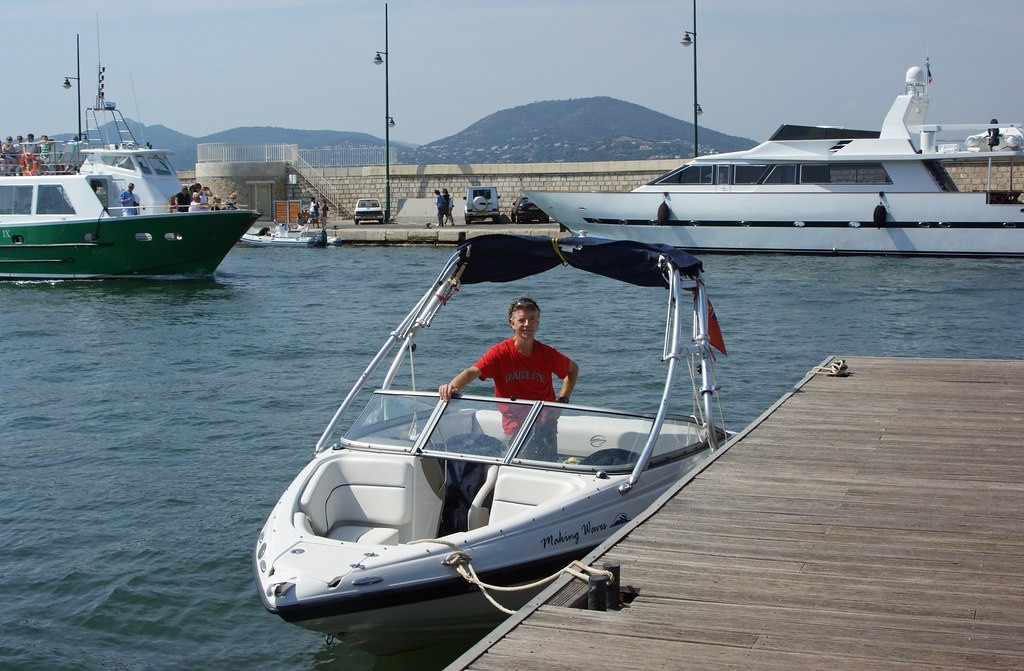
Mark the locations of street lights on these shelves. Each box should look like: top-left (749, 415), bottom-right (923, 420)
top-left (680, 32), bottom-right (703, 156)
top-left (63, 77), bottom-right (82, 142)
top-left (374, 51), bottom-right (397, 220)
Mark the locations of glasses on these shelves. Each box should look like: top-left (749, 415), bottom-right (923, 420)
top-left (510, 298), bottom-right (537, 315)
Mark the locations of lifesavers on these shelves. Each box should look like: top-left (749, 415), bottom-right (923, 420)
top-left (20, 153), bottom-right (38, 175)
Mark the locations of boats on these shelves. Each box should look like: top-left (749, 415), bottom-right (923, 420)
top-left (240, 216), bottom-right (342, 247)
top-left (514, 63), bottom-right (1024, 259)
top-left (251, 232), bottom-right (744, 657)
top-left (0, 66), bottom-right (265, 282)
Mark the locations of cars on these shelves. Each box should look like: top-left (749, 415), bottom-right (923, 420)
top-left (351, 199), bottom-right (385, 225)
top-left (514, 196), bottom-right (549, 223)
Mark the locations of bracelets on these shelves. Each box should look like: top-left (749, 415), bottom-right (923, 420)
top-left (557, 397), bottom-right (569, 403)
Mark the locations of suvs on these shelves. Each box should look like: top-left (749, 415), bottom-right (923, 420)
top-left (463, 185), bottom-right (502, 224)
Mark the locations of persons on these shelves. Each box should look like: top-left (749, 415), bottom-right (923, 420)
top-left (0, 135), bottom-right (53, 176)
top-left (434, 188), bottom-right (455, 227)
top-left (165, 182), bottom-right (241, 212)
top-left (439, 298), bottom-right (580, 462)
top-left (308, 197), bottom-right (320, 229)
top-left (119, 182), bottom-right (139, 216)
